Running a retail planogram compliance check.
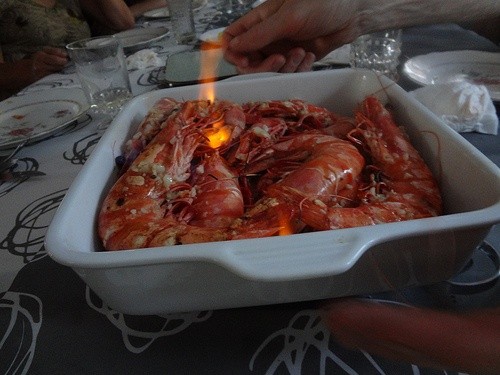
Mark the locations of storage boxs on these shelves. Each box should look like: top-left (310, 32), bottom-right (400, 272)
top-left (44, 68), bottom-right (500, 316)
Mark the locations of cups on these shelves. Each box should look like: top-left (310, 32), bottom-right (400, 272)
top-left (350, 26), bottom-right (403, 79)
top-left (166, 0), bottom-right (196, 45)
top-left (66, 36), bottom-right (134, 117)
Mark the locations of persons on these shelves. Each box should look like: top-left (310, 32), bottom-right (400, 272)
top-left (0, 0), bottom-right (136, 102)
top-left (222, 1), bottom-right (500, 74)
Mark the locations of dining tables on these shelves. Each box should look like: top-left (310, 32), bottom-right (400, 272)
top-left (0, 0), bottom-right (500, 375)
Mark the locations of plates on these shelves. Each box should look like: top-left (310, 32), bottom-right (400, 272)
top-left (0, 88), bottom-right (90, 149)
top-left (164, 48), bottom-right (238, 82)
top-left (43, 68), bottom-right (500, 316)
top-left (143, 0), bottom-right (207, 18)
top-left (199, 26), bottom-right (226, 46)
top-left (402, 49), bottom-right (500, 100)
top-left (111, 26), bottom-right (170, 52)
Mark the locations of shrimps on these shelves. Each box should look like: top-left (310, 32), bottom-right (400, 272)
top-left (98, 96), bottom-right (444, 251)
